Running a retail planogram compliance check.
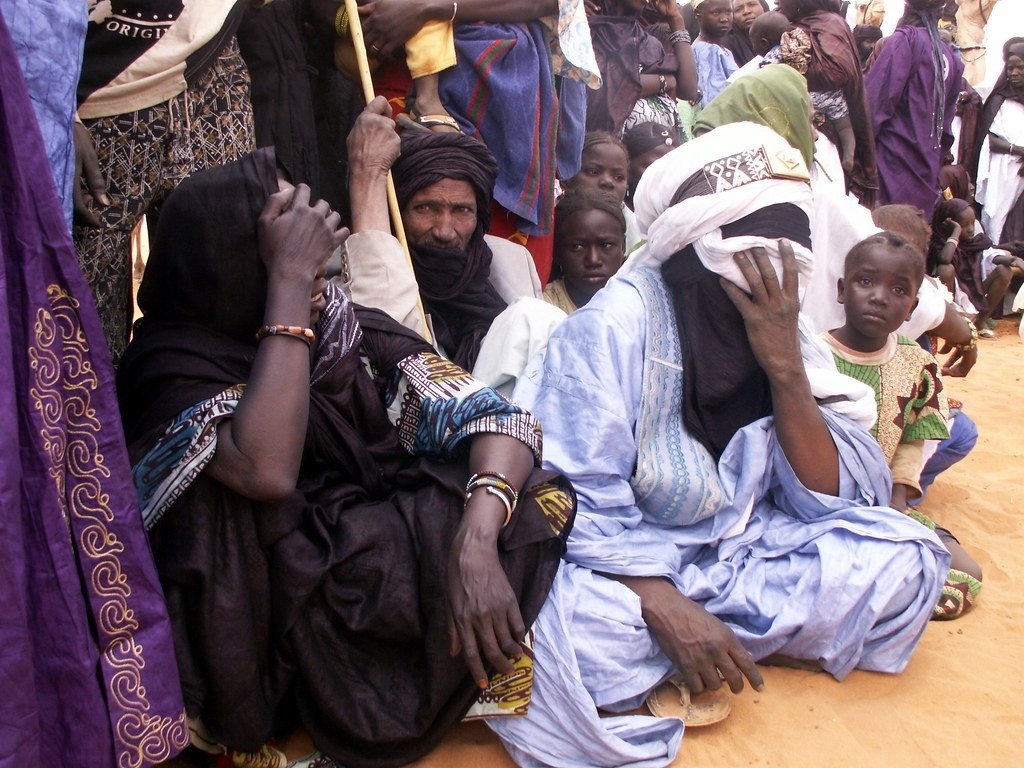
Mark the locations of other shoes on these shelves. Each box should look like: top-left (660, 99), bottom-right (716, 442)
top-left (986, 318), bottom-right (996, 329)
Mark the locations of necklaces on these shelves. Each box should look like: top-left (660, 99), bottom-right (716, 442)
top-left (699, 34), bottom-right (729, 55)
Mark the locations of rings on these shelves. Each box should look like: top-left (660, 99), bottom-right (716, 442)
top-left (371, 45), bottom-right (380, 51)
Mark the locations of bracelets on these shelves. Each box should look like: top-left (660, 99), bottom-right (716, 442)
top-left (953, 315), bottom-right (979, 351)
top-left (254, 324), bottom-right (315, 347)
top-left (465, 478), bottom-right (516, 511)
top-left (334, 4), bottom-right (346, 38)
top-left (467, 471), bottom-right (518, 508)
top-left (659, 73), bottom-right (668, 96)
top-left (448, 1), bottom-right (458, 23)
top-left (1008, 143), bottom-right (1015, 153)
top-left (341, 12), bottom-right (349, 35)
top-left (669, 30), bottom-right (692, 46)
top-left (463, 486), bottom-right (512, 526)
top-left (948, 237), bottom-right (958, 241)
top-left (946, 238), bottom-right (958, 247)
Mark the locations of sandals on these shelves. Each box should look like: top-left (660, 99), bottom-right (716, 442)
top-left (408, 100), bottom-right (465, 136)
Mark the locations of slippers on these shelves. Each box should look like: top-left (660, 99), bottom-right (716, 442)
top-left (973, 319), bottom-right (996, 340)
top-left (647, 672), bottom-right (730, 727)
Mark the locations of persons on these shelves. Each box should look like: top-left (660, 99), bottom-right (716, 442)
top-left (1, 0), bottom-right (1024, 767)
